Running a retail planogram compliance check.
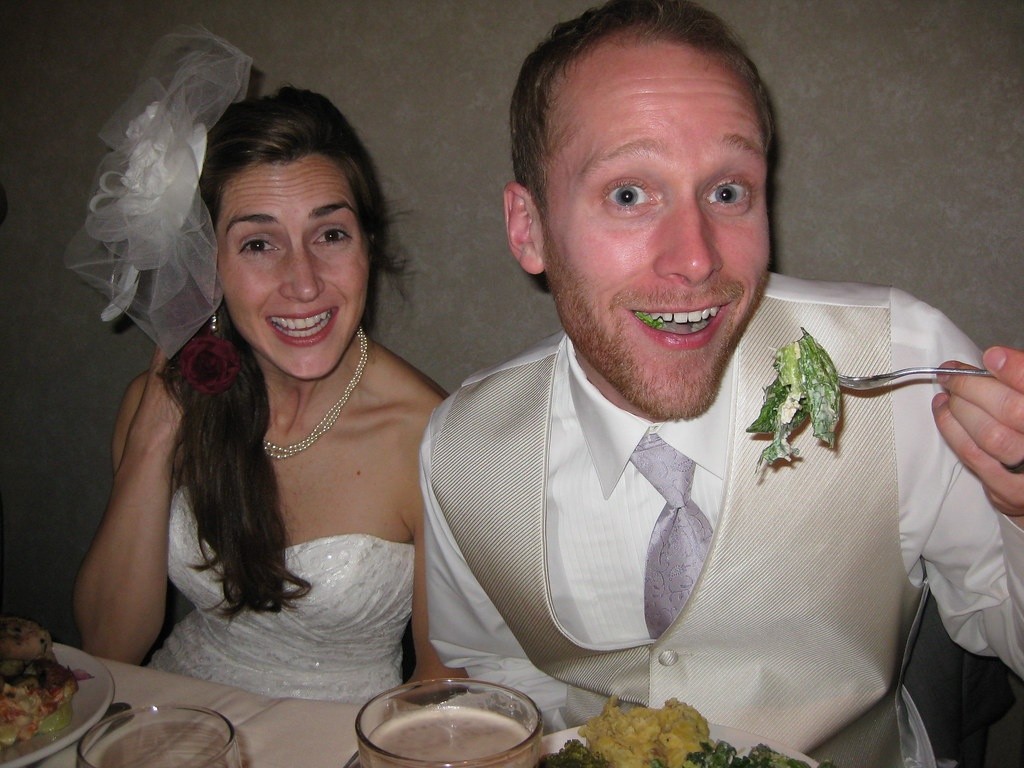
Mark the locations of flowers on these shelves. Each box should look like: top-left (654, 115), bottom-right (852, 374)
top-left (61, 20), bottom-right (254, 394)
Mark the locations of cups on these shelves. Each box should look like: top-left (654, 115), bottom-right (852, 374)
top-left (76, 705), bottom-right (242, 767)
top-left (353, 676), bottom-right (543, 768)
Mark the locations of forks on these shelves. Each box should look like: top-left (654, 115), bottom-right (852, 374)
top-left (838, 366), bottom-right (990, 390)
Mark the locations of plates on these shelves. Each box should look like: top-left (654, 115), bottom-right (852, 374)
top-left (0, 642), bottom-right (115, 768)
top-left (540, 719), bottom-right (820, 768)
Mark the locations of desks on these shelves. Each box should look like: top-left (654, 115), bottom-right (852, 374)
top-left (38, 658), bottom-right (389, 767)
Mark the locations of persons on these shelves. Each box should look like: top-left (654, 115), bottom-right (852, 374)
top-left (61, 29), bottom-right (469, 705)
top-left (418, 0), bottom-right (1024, 768)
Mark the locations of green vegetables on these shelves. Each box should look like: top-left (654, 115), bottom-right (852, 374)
top-left (635, 314), bottom-right (662, 330)
top-left (744, 328), bottom-right (842, 471)
top-left (532, 739), bottom-right (837, 768)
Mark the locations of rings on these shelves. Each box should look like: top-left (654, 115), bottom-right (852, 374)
top-left (1001, 460), bottom-right (1024, 473)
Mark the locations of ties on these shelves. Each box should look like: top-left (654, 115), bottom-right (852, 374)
top-left (628, 432), bottom-right (714, 639)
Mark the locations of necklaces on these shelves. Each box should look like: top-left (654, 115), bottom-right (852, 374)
top-left (261, 325), bottom-right (368, 460)
